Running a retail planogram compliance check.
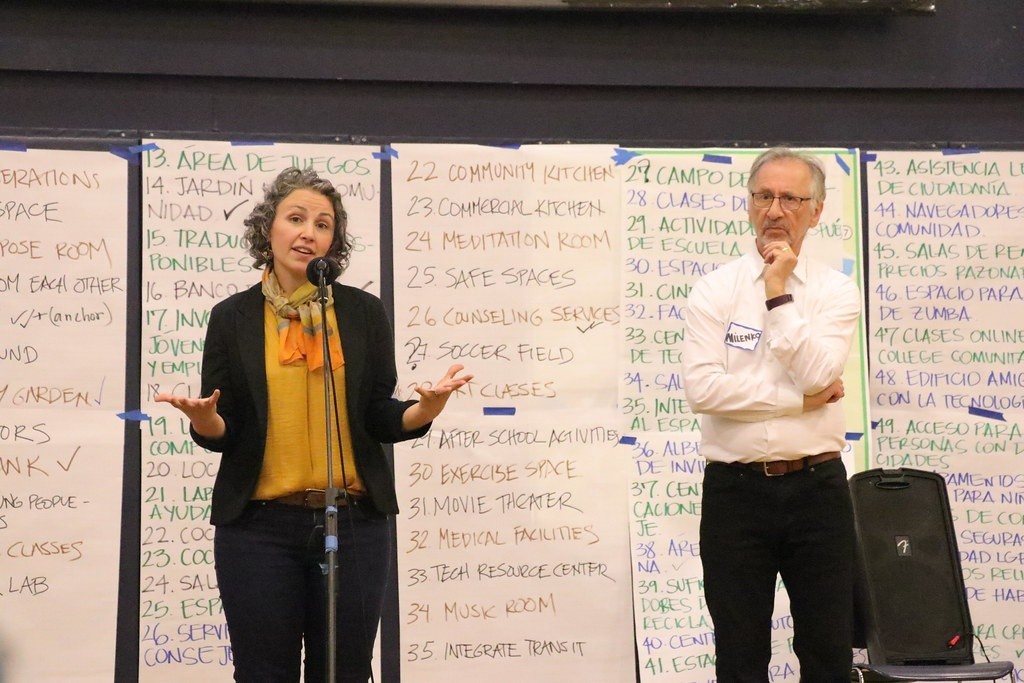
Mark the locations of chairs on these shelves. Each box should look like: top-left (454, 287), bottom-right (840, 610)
top-left (840, 467), bottom-right (1015, 683)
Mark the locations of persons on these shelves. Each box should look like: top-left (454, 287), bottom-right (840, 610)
top-left (681, 146), bottom-right (860, 683)
top-left (155, 164), bottom-right (475, 683)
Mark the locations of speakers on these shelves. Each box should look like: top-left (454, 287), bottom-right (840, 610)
top-left (852, 467), bottom-right (973, 664)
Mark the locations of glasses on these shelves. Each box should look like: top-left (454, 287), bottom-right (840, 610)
top-left (752, 192), bottom-right (812, 212)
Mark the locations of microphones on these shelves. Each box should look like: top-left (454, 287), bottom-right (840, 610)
top-left (305, 256), bottom-right (335, 286)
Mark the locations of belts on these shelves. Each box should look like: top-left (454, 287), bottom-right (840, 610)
top-left (250, 488), bottom-right (368, 509)
top-left (712, 451), bottom-right (841, 477)
